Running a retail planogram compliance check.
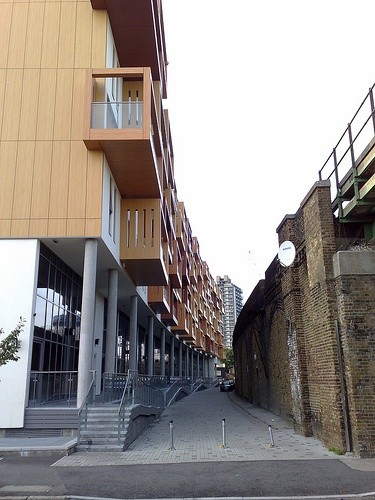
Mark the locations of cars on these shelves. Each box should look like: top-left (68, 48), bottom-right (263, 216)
top-left (217, 376), bottom-right (236, 392)
